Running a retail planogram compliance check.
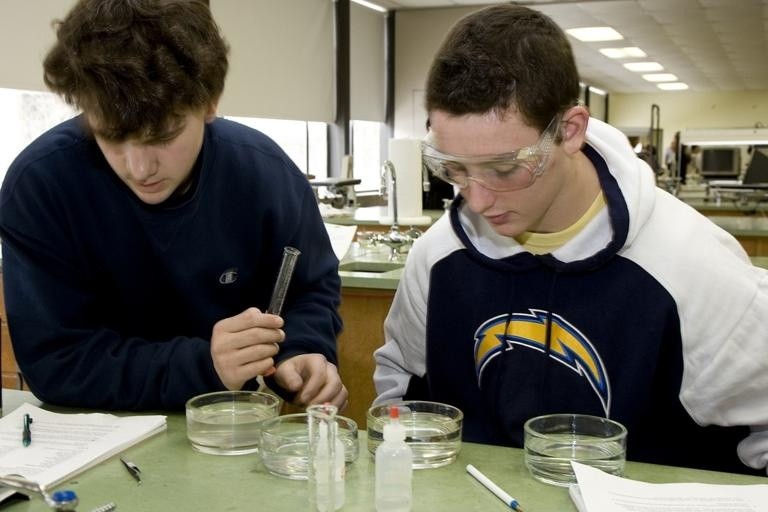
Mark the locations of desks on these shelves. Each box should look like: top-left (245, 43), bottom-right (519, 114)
top-left (325, 183), bottom-right (767, 427)
top-left (0, 385), bottom-right (767, 512)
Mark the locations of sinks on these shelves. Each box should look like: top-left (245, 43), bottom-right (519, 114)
top-left (338, 260), bottom-right (406, 279)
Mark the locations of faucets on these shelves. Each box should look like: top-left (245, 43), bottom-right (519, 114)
top-left (379, 160), bottom-right (403, 260)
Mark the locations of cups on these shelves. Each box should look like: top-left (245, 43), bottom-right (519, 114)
top-left (356, 231), bottom-right (385, 249)
top-left (524, 413), bottom-right (627, 489)
top-left (185, 388), bottom-right (280, 456)
top-left (366, 399), bottom-right (465, 471)
top-left (259, 410), bottom-right (359, 481)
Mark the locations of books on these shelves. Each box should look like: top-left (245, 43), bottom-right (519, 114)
top-left (569, 462), bottom-right (767, 512)
top-left (0, 402), bottom-right (167, 493)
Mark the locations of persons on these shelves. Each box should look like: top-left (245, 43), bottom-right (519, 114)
top-left (373, 4), bottom-right (768, 483)
top-left (1, 0), bottom-right (349, 414)
top-left (637, 135), bottom-right (702, 184)
top-left (422, 116), bottom-right (454, 210)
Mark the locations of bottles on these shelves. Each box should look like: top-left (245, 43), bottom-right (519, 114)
top-left (268, 247), bottom-right (301, 317)
top-left (303, 403), bottom-right (414, 511)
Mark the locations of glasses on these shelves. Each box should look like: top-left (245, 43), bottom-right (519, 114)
top-left (419, 108), bottom-right (563, 192)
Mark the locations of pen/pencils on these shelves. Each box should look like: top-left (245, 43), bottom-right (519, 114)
top-left (465, 463), bottom-right (525, 512)
top-left (23, 414), bottom-right (32, 447)
